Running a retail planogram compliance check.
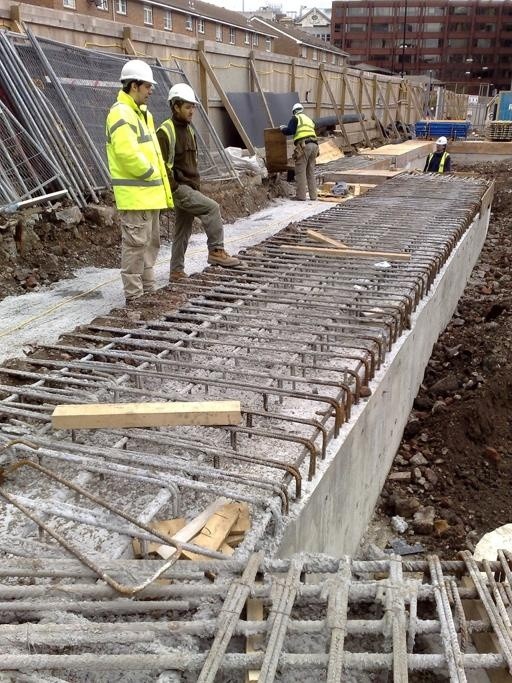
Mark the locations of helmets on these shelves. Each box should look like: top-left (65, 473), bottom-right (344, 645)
top-left (120, 60), bottom-right (158, 84)
top-left (435, 136), bottom-right (447, 144)
top-left (292, 103), bottom-right (303, 111)
top-left (168, 83), bottom-right (199, 104)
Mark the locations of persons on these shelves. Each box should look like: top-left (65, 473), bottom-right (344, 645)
top-left (156, 82), bottom-right (241, 282)
top-left (422, 135), bottom-right (451, 173)
top-left (279, 102), bottom-right (321, 200)
top-left (104, 59), bottom-right (176, 303)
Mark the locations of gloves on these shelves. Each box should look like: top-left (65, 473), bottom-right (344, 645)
top-left (280, 125), bottom-right (288, 130)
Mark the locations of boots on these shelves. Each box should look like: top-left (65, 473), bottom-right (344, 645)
top-left (169, 272), bottom-right (191, 282)
top-left (207, 248), bottom-right (240, 266)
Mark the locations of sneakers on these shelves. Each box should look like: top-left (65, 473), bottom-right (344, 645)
top-left (290, 196), bottom-right (316, 201)
